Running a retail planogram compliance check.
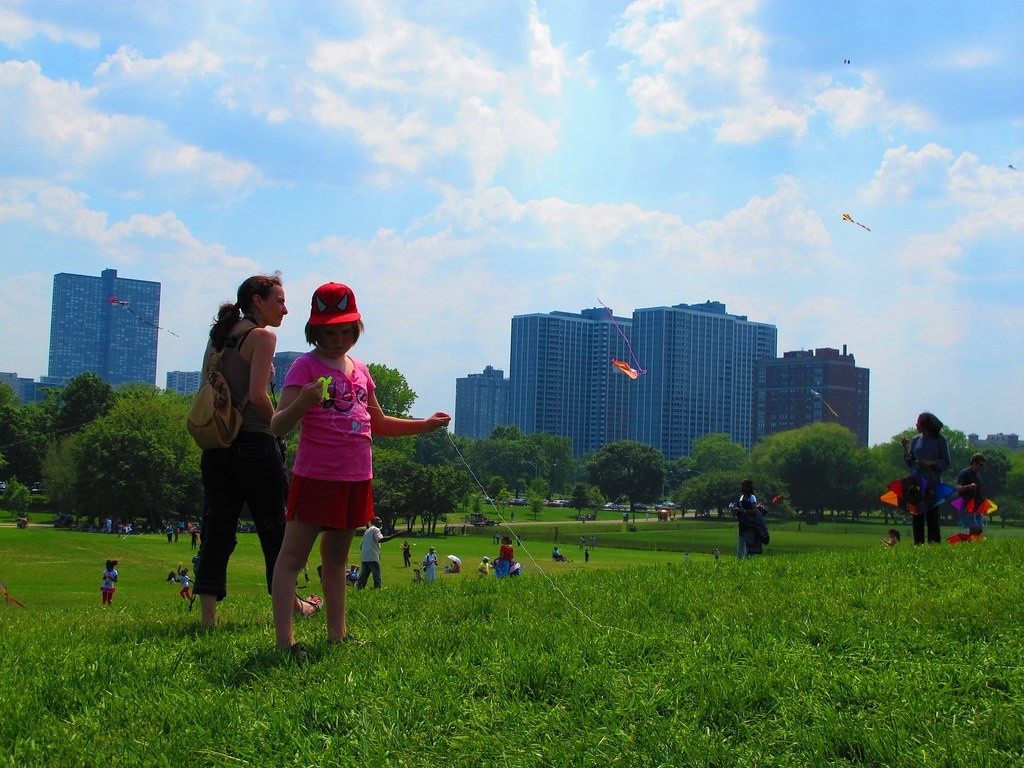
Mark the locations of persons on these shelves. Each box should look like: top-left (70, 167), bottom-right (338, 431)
top-left (901, 412), bottom-right (951, 546)
top-left (729, 479), bottom-right (759, 561)
top-left (880, 530), bottom-right (901, 548)
top-left (301, 512), bottom-right (522, 592)
top-left (552, 503), bottom-right (721, 563)
top-left (192, 271), bottom-right (324, 630)
top-left (956, 453), bottom-right (990, 536)
top-left (16, 512), bottom-right (258, 605)
top-left (271, 282), bottom-right (450, 656)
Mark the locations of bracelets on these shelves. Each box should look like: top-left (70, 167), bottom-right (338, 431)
top-left (743, 510), bottom-right (745, 513)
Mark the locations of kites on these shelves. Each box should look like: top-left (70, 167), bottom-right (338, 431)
top-left (107, 294), bottom-right (179, 338)
top-left (843, 60), bottom-right (852, 64)
top-left (1008, 165), bottom-right (1017, 170)
top-left (842, 213), bottom-right (872, 232)
top-left (597, 299), bottom-right (647, 380)
top-left (881, 472), bottom-right (998, 546)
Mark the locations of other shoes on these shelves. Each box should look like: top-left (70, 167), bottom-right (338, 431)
top-left (327, 633), bottom-right (357, 644)
top-left (286, 643), bottom-right (315, 662)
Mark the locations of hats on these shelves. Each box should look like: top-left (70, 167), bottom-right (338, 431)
top-left (429, 547), bottom-right (436, 553)
top-left (309, 281), bottom-right (361, 325)
top-left (482, 556), bottom-right (490, 560)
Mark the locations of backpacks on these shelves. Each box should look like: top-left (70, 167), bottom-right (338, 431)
top-left (423, 554), bottom-right (436, 572)
top-left (186, 325), bottom-right (257, 450)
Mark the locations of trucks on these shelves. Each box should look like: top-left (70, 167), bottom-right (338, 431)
top-left (657, 508), bottom-right (675, 521)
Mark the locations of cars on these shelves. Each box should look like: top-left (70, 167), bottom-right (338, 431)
top-left (483, 498), bottom-right (677, 511)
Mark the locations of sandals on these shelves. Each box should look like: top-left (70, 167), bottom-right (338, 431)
top-left (300, 594), bottom-right (324, 618)
